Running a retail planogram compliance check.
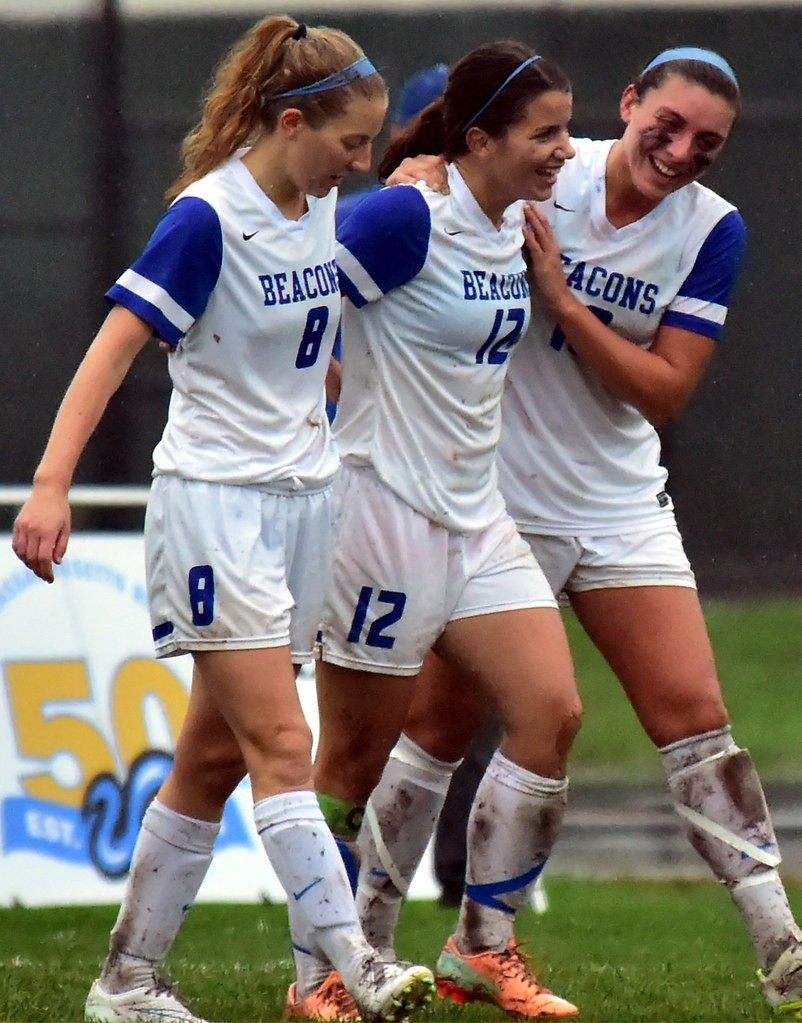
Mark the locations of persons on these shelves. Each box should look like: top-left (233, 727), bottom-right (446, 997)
top-left (356, 46), bottom-right (802, 1023)
top-left (154, 39), bottom-right (585, 1023)
top-left (11, 16), bottom-right (435, 1023)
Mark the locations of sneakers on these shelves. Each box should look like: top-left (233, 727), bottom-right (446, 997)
top-left (344, 947), bottom-right (435, 1023)
top-left (81, 979), bottom-right (209, 1023)
top-left (281, 970), bottom-right (362, 1023)
top-left (434, 934), bottom-right (580, 1023)
top-left (759, 926), bottom-right (802, 1013)
